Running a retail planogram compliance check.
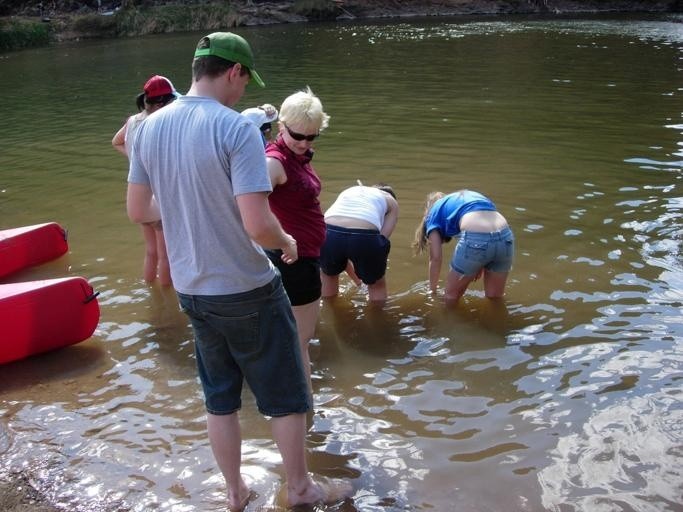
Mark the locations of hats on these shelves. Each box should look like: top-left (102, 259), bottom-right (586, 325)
top-left (143, 76), bottom-right (182, 97)
top-left (195, 33), bottom-right (265, 88)
top-left (238, 103), bottom-right (280, 130)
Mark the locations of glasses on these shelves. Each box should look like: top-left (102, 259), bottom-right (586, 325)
top-left (283, 123), bottom-right (319, 142)
top-left (260, 123), bottom-right (272, 132)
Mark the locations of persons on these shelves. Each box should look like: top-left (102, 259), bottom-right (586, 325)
top-left (413, 191), bottom-right (515, 300)
top-left (126, 32), bottom-right (352, 512)
top-left (241, 104), bottom-right (277, 144)
top-left (111, 76), bottom-right (182, 286)
top-left (320, 186), bottom-right (399, 299)
top-left (265, 86), bottom-right (330, 394)
